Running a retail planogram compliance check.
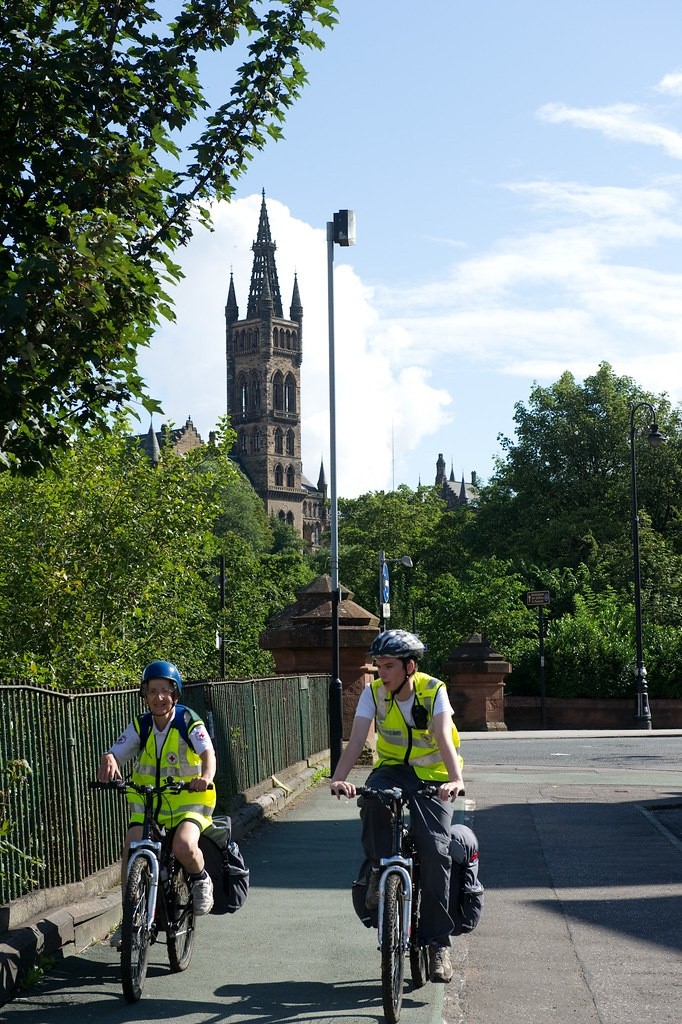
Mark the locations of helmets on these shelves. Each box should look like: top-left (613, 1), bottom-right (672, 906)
top-left (138, 662), bottom-right (183, 696)
top-left (370, 629), bottom-right (426, 658)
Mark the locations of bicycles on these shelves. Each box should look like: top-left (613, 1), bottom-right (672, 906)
top-left (87, 778), bottom-right (215, 1002)
top-left (331, 789), bottom-right (466, 1024)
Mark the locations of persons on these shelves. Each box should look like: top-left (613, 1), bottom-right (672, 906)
top-left (329, 629), bottom-right (465, 984)
top-left (98, 661), bottom-right (217, 948)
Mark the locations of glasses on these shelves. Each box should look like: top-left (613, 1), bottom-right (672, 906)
top-left (145, 688), bottom-right (175, 695)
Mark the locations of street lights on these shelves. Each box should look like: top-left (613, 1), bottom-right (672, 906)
top-left (378, 550), bottom-right (413, 634)
top-left (326, 210), bottom-right (356, 780)
top-left (630, 402), bottom-right (666, 731)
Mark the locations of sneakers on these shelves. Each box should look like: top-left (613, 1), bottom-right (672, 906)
top-left (110, 921), bottom-right (122, 947)
top-left (191, 870), bottom-right (214, 914)
top-left (429, 942), bottom-right (455, 983)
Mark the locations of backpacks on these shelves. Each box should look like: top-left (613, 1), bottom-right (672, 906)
top-left (197, 815), bottom-right (249, 916)
top-left (352, 858), bottom-right (379, 927)
top-left (450, 824), bottom-right (484, 935)
